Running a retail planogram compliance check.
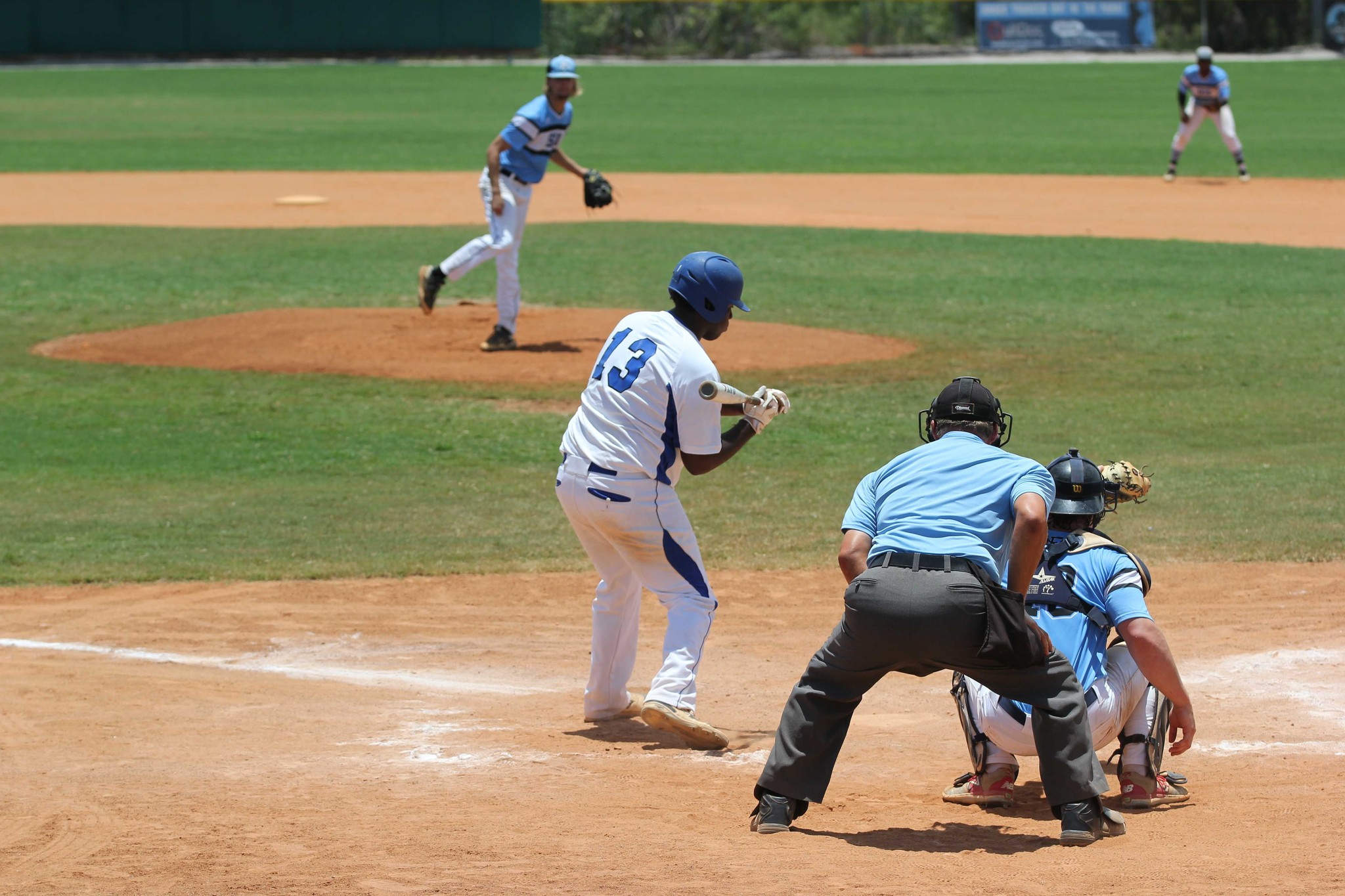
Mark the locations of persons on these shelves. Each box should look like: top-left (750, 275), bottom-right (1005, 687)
top-left (417, 52), bottom-right (614, 351)
top-left (748, 376), bottom-right (1126, 848)
top-left (941, 447), bottom-right (1196, 810)
top-left (1164, 45), bottom-right (1250, 185)
top-left (553, 249), bottom-right (791, 751)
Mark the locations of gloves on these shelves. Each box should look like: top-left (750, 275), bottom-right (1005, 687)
top-left (742, 385), bottom-right (790, 435)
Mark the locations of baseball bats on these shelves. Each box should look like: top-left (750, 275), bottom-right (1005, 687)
top-left (699, 381), bottom-right (765, 414)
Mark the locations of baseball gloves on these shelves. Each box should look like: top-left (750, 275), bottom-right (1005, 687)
top-left (1095, 457), bottom-right (1152, 505)
top-left (583, 169), bottom-right (612, 208)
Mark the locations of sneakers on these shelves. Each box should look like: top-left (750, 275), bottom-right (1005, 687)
top-left (480, 328), bottom-right (515, 350)
top-left (640, 700), bottom-right (729, 748)
top-left (419, 264), bottom-right (440, 314)
top-left (749, 790), bottom-right (796, 832)
top-left (1059, 801), bottom-right (1127, 846)
top-left (585, 693), bottom-right (647, 722)
top-left (941, 767), bottom-right (1015, 807)
top-left (1118, 771), bottom-right (1190, 805)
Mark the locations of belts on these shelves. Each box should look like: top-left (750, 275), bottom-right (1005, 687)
top-left (502, 169), bottom-right (526, 185)
top-left (869, 552), bottom-right (973, 573)
top-left (562, 452), bottom-right (617, 475)
top-left (998, 687), bottom-right (1098, 727)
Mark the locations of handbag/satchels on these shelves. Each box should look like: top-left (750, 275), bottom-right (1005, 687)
top-left (966, 559), bottom-right (1043, 666)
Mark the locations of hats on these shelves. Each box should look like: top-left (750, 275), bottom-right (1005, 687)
top-left (546, 54), bottom-right (578, 78)
top-left (1197, 47), bottom-right (1214, 62)
top-left (933, 380), bottom-right (999, 424)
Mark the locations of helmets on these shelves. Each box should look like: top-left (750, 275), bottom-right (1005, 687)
top-left (667, 251), bottom-right (750, 322)
top-left (1049, 458), bottom-right (1104, 514)
